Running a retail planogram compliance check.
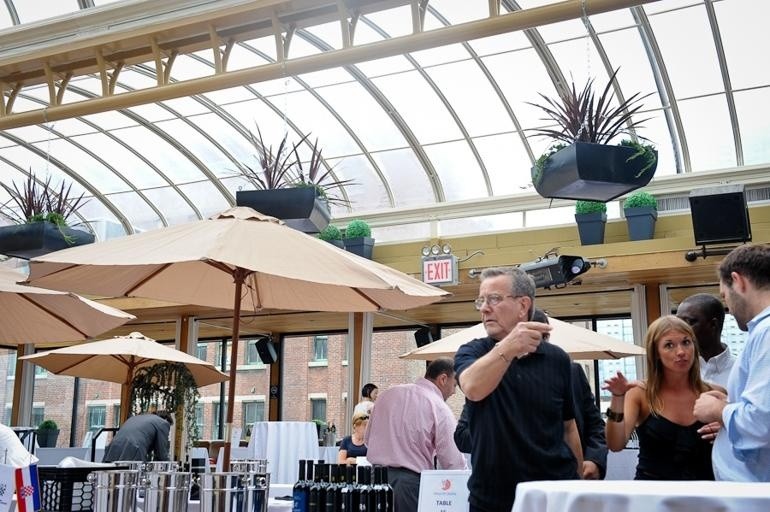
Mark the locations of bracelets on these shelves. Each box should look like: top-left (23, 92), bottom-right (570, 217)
top-left (606, 408), bottom-right (624, 422)
top-left (495, 343), bottom-right (512, 367)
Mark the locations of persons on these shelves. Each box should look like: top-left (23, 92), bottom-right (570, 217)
top-left (353, 383), bottom-right (379, 419)
top-left (339, 413), bottom-right (368, 465)
top-left (0, 423), bottom-right (40, 469)
top-left (692, 241), bottom-right (769, 482)
top-left (452, 267), bottom-right (584, 512)
top-left (453, 308), bottom-right (608, 480)
top-left (364, 356), bottom-right (470, 512)
top-left (102, 408), bottom-right (173, 463)
top-left (677, 292), bottom-right (738, 395)
top-left (600, 315), bottom-right (726, 480)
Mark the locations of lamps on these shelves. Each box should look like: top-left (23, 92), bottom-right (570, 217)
top-left (568, 255), bottom-right (609, 277)
top-left (419, 242), bottom-right (453, 258)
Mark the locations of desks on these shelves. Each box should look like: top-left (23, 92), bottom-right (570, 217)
top-left (33, 445), bottom-right (101, 470)
top-left (243, 419), bottom-right (322, 485)
top-left (509, 477), bottom-right (770, 512)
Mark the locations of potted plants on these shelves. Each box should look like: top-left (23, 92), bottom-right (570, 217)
top-left (343, 218), bottom-right (376, 261)
top-left (221, 118), bottom-right (365, 235)
top-left (317, 225), bottom-right (346, 250)
top-left (516, 61), bottom-right (663, 207)
top-left (572, 198), bottom-right (611, 246)
top-left (34, 417), bottom-right (63, 447)
top-left (1, 161), bottom-right (102, 261)
top-left (621, 190), bottom-right (660, 241)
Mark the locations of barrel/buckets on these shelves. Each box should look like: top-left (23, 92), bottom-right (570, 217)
top-left (90, 459), bottom-right (272, 512)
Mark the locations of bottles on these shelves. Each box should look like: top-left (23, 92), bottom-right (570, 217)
top-left (293, 457), bottom-right (395, 512)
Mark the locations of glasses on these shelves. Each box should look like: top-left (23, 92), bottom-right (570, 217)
top-left (354, 415), bottom-right (369, 423)
top-left (475, 295), bottom-right (524, 311)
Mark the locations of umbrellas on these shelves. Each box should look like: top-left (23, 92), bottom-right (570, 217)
top-left (0, 282), bottom-right (138, 347)
top-left (16, 330), bottom-right (234, 427)
top-left (16, 203), bottom-right (451, 471)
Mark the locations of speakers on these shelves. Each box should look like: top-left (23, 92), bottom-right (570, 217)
top-left (689, 185), bottom-right (751, 245)
top-left (414, 329), bottom-right (433, 348)
top-left (255, 338), bottom-right (278, 365)
